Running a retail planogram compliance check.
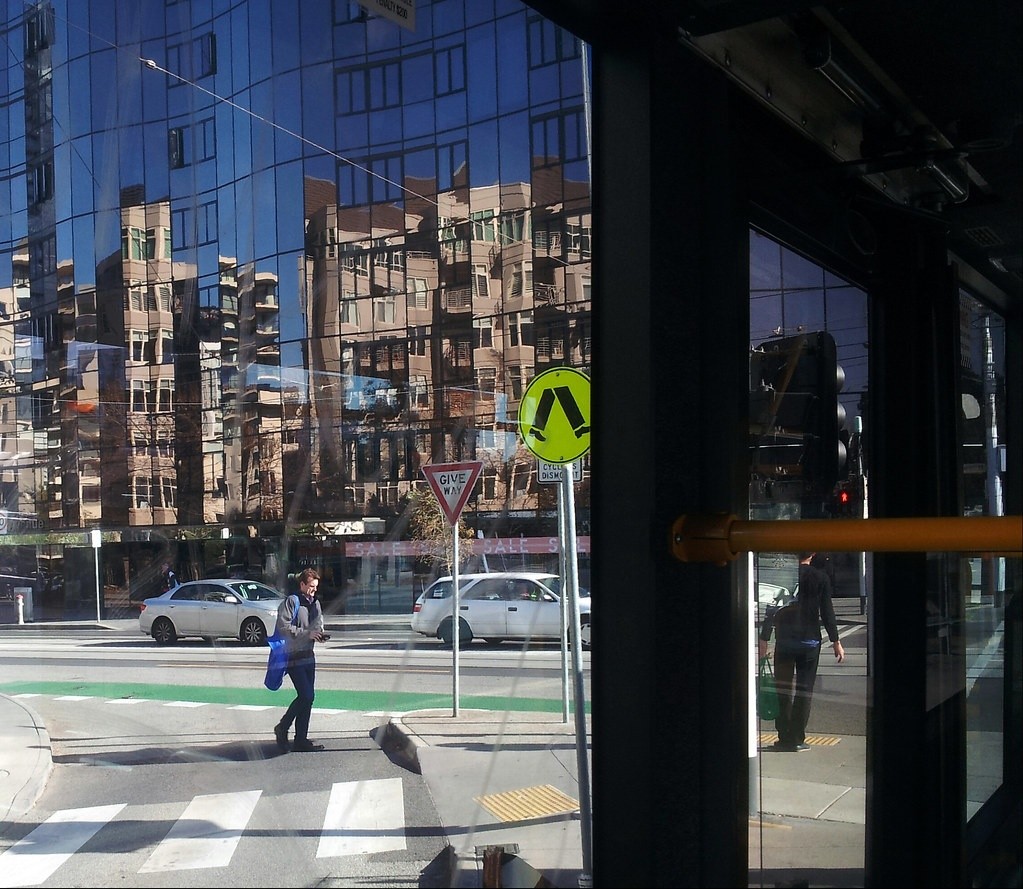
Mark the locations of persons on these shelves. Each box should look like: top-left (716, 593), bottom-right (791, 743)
top-left (160, 562), bottom-right (180, 591)
top-left (273, 568), bottom-right (330, 751)
top-left (758, 548), bottom-right (845, 752)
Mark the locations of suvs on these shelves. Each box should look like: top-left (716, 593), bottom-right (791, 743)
top-left (411, 573), bottom-right (592, 650)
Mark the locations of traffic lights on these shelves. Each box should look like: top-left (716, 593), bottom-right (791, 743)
top-left (840, 479), bottom-right (859, 505)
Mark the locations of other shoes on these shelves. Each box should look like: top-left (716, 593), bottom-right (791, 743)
top-left (774, 742), bottom-right (792, 750)
top-left (793, 743), bottom-right (811, 752)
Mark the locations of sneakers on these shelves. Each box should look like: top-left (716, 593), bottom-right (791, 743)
top-left (293, 740), bottom-right (324, 752)
top-left (274, 724), bottom-right (291, 753)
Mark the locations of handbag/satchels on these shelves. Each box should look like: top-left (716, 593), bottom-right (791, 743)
top-left (263, 637), bottom-right (288, 691)
top-left (756, 657), bottom-right (782, 720)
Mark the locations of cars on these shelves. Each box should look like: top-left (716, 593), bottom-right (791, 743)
top-left (0, 561), bottom-right (65, 604)
top-left (209, 564), bottom-right (265, 584)
top-left (757, 582), bottom-right (789, 628)
top-left (137, 579), bottom-right (292, 648)
top-left (131, 571), bottom-right (188, 599)
top-left (329, 558), bottom-right (381, 593)
